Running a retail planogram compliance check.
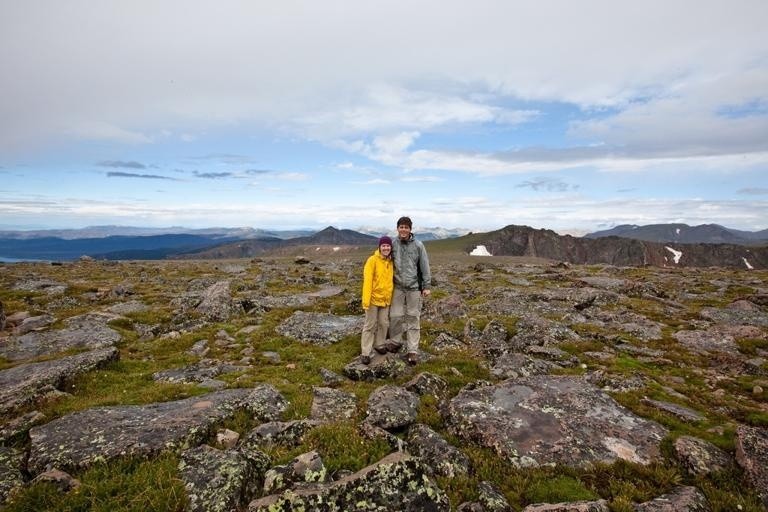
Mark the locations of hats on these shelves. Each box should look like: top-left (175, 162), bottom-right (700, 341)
top-left (397, 217), bottom-right (411, 229)
top-left (379, 237), bottom-right (392, 246)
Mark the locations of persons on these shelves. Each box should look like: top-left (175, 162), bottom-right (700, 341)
top-left (386, 217), bottom-right (432, 364)
top-left (360, 236), bottom-right (395, 365)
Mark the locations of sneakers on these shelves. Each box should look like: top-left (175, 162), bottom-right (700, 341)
top-left (408, 353), bottom-right (417, 363)
top-left (374, 343), bottom-right (401, 354)
top-left (362, 356), bottom-right (370, 364)
